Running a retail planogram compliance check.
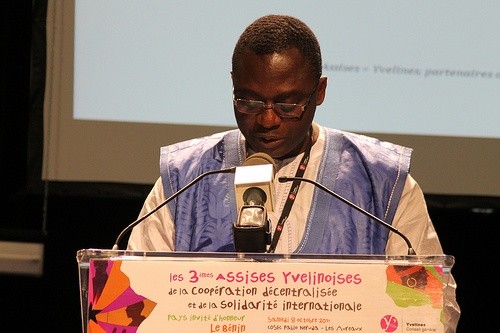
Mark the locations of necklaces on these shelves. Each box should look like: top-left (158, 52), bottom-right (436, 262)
top-left (237, 125), bottom-right (332, 257)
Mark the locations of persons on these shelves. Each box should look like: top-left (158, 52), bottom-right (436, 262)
top-left (123, 12), bottom-right (464, 333)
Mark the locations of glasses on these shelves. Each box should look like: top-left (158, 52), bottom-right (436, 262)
top-left (233, 74), bottom-right (320, 119)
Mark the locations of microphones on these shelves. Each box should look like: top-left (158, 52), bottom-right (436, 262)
top-left (112, 164), bottom-right (236, 250)
top-left (278, 176), bottom-right (418, 256)
top-left (236, 152), bottom-right (276, 230)
